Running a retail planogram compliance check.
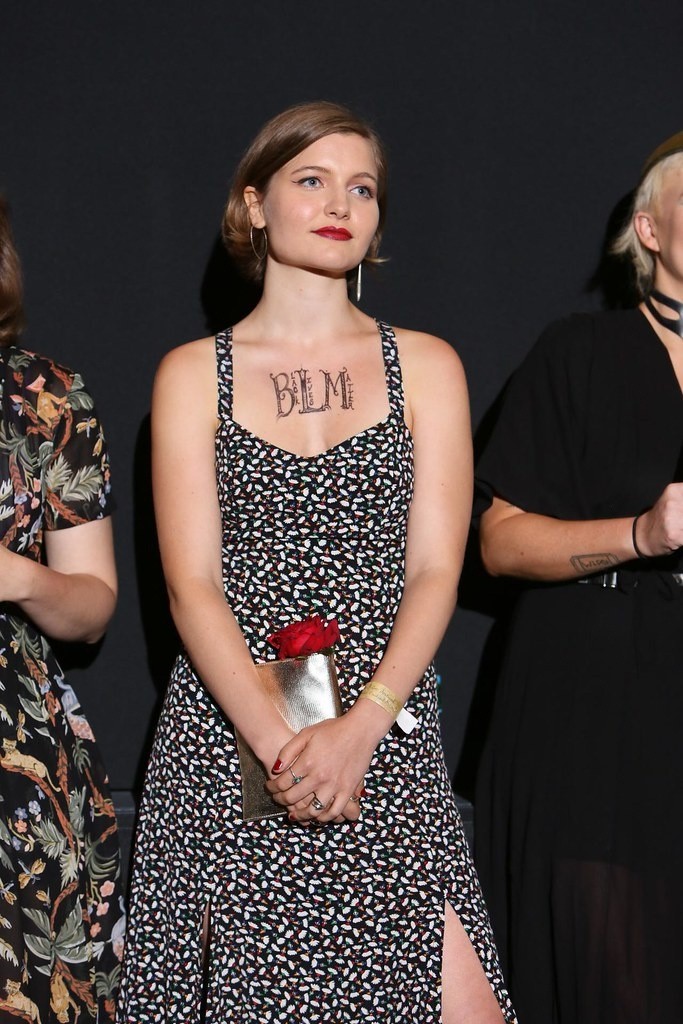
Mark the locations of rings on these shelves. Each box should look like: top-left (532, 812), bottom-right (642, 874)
top-left (312, 798), bottom-right (325, 810)
top-left (349, 794), bottom-right (359, 802)
top-left (290, 768), bottom-right (302, 784)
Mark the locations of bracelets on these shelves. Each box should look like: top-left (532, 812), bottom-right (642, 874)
top-left (358, 682), bottom-right (419, 735)
top-left (632, 516), bottom-right (654, 559)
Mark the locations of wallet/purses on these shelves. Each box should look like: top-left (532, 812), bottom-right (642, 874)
top-left (235, 652), bottom-right (342, 821)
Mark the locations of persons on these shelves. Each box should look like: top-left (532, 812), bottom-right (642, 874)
top-left (451, 149), bottom-right (683, 1024)
top-left (0, 204), bottom-right (126, 1024)
top-left (115, 104), bottom-right (522, 1024)
